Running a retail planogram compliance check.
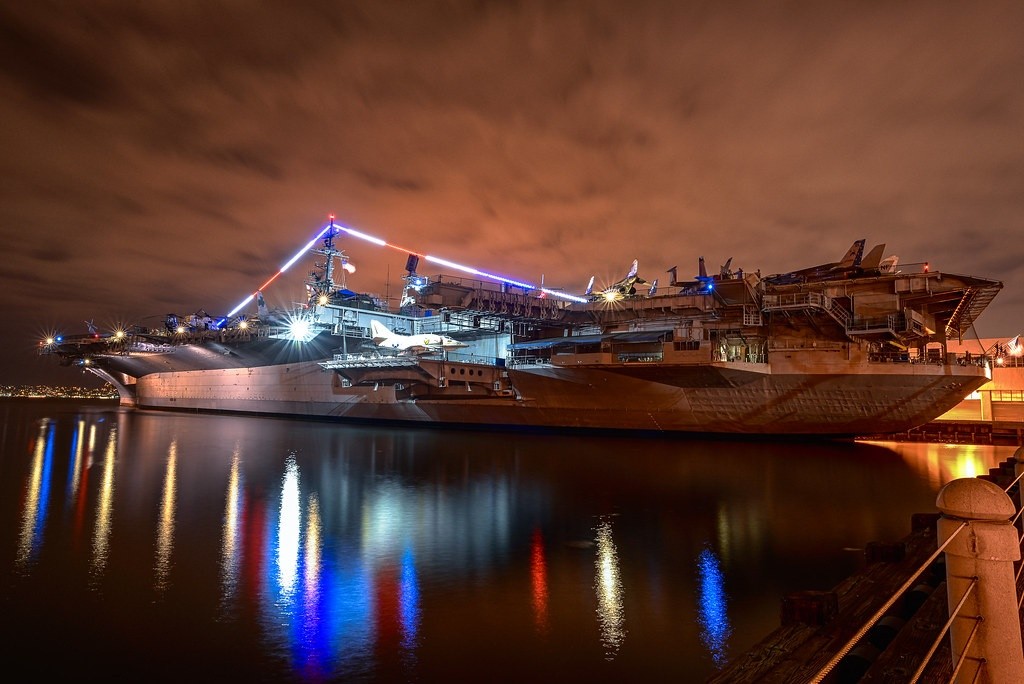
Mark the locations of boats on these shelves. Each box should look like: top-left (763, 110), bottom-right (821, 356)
top-left (41, 211), bottom-right (1004, 443)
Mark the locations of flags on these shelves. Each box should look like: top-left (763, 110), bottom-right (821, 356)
top-left (995, 344), bottom-right (998, 357)
top-left (342, 259), bottom-right (356, 275)
top-left (1003, 349), bottom-right (1008, 357)
top-left (1007, 336), bottom-right (1018, 351)
top-left (999, 346), bottom-right (1003, 356)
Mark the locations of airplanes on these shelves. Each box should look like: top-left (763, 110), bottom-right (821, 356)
top-left (582, 239), bottom-right (891, 303)
